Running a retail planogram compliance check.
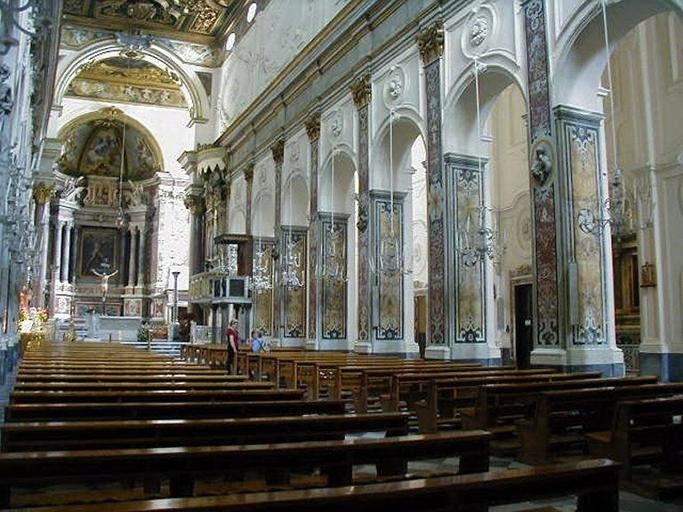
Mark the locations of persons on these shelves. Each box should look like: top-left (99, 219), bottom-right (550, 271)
top-left (0, 59), bottom-right (15, 132)
top-left (64, 176), bottom-right (78, 198)
top-left (131, 182), bottom-right (143, 197)
top-left (225, 318), bottom-right (238, 373)
top-left (91, 268), bottom-right (118, 301)
top-left (245, 330), bottom-right (257, 346)
top-left (250, 328), bottom-right (269, 352)
